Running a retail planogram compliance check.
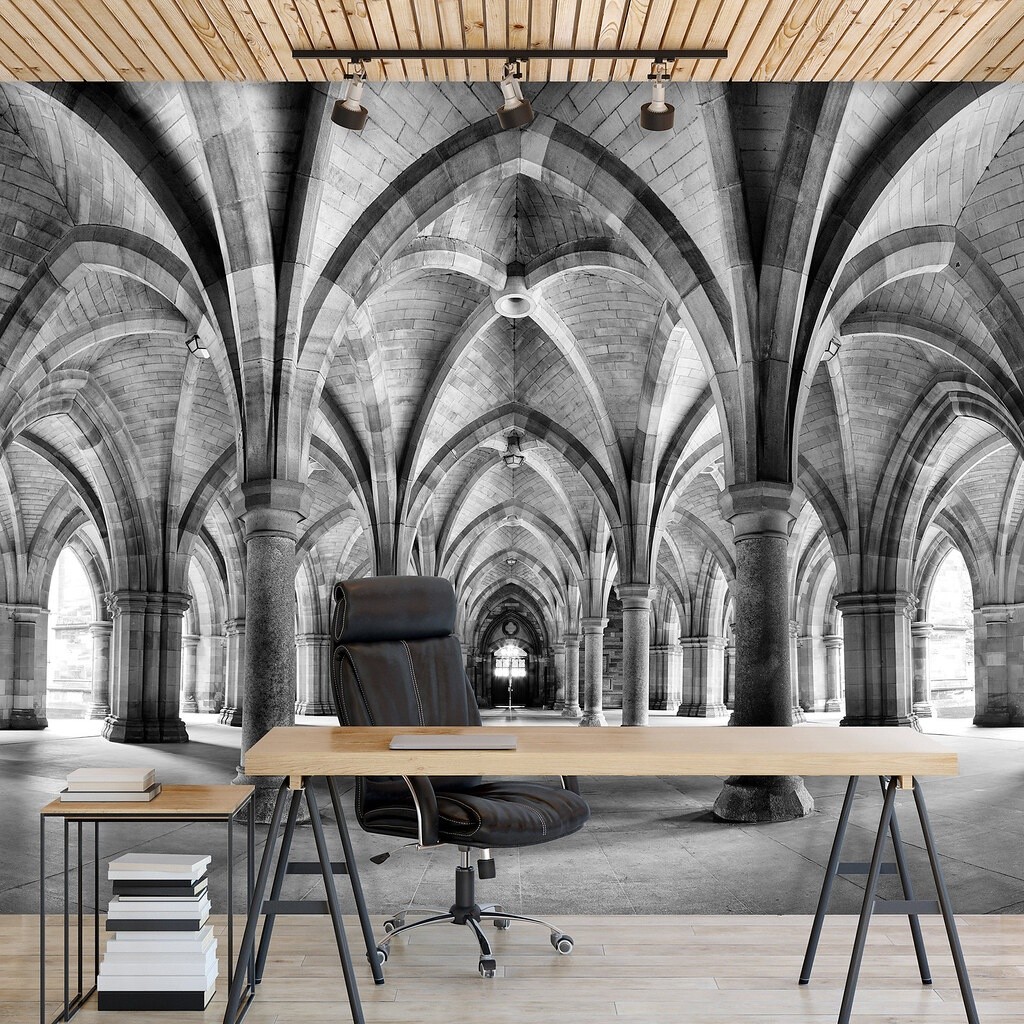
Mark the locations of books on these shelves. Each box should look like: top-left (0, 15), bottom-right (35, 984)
top-left (59, 766), bottom-right (162, 803)
top-left (388, 732), bottom-right (520, 751)
top-left (96, 854), bottom-right (219, 1011)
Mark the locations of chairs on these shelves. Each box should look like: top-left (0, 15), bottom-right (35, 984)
top-left (328, 576), bottom-right (587, 978)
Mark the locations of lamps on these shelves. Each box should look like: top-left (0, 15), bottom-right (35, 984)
top-left (329, 63), bottom-right (372, 131)
top-left (184, 329), bottom-right (210, 358)
top-left (820, 328), bottom-right (840, 364)
top-left (502, 431), bottom-right (522, 467)
top-left (493, 61), bottom-right (534, 130)
top-left (639, 74), bottom-right (676, 131)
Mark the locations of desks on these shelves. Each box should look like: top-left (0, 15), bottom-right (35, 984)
top-left (239, 723), bottom-right (980, 1024)
top-left (40, 784), bottom-right (256, 1023)
